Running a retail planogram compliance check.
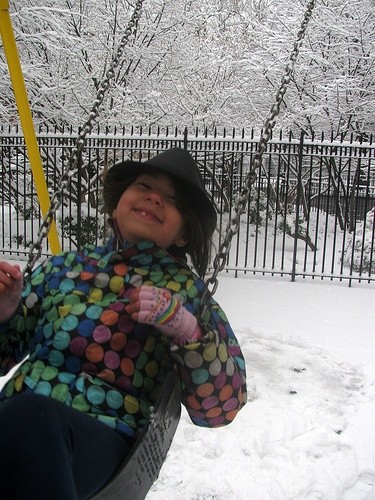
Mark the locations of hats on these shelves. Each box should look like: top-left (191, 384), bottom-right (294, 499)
top-left (103, 147), bottom-right (217, 256)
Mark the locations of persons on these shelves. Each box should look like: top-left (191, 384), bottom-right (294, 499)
top-left (0, 147), bottom-right (246, 500)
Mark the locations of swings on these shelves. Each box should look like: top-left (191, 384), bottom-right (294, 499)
top-left (17, 1), bottom-right (317, 500)
